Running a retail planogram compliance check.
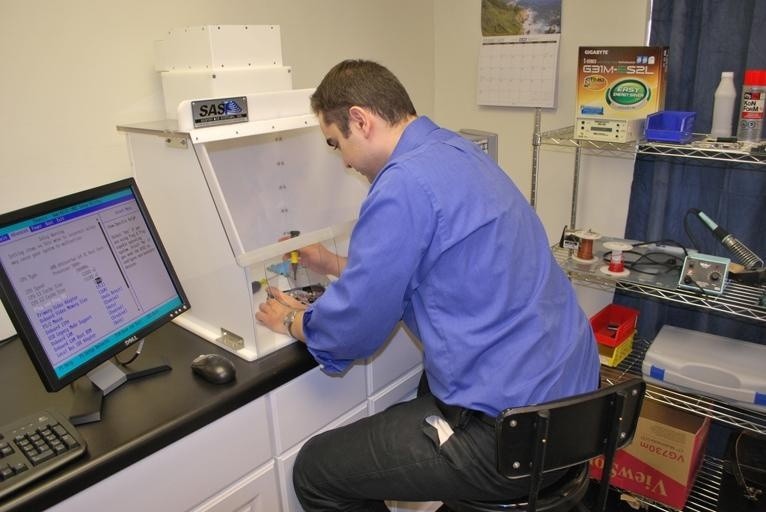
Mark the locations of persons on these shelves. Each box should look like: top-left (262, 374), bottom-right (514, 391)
top-left (255, 60), bottom-right (601, 512)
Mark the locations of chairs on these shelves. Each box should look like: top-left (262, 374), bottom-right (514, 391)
top-left (439, 375), bottom-right (647, 512)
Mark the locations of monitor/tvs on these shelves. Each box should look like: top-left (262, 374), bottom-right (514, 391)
top-left (0, 178), bottom-right (190, 424)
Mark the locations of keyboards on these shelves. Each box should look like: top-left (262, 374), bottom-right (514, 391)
top-left (1, 404), bottom-right (87, 499)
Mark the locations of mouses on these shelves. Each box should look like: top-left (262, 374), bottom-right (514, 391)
top-left (192, 354), bottom-right (238, 385)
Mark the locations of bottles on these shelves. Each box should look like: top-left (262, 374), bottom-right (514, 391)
top-left (711, 70), bottom-right (766, 143)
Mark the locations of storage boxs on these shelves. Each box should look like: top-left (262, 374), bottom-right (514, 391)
top-left (587, 397), bottom-right (715, 511)
top-left (574, 43), bottom-right (670, 120)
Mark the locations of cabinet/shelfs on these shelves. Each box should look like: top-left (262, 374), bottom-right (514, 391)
top-left (262, 341), bottom-right (373, 512)
top-left (0, 376), bottom-right (283, 512)
top-left (366, 316), bottom-right (428, 417)
top-left (527, 102), bottom-right (766, 512)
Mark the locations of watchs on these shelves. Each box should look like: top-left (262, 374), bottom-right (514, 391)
top-left (283, 308), bottom-right (303, 339)
